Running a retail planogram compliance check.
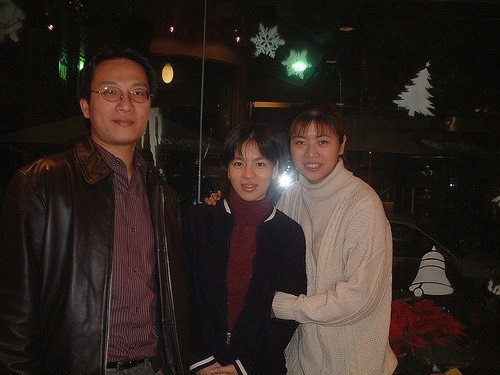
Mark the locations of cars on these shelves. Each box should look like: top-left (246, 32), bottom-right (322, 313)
top-left (386, 214), bottom-right (460, 298)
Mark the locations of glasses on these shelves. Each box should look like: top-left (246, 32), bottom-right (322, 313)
top-left (90, 86), bottom-right (153, 103)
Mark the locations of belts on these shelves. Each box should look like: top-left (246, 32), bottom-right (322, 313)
top-left (107, 358), bottom-right (144, 371)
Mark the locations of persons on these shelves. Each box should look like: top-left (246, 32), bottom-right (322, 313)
top-left (180, 120), bottom-right (308, 375)
top-left (204, 109), bottom-right (398, 375)
top-left (0, 48), bottom-right (188, 374)
